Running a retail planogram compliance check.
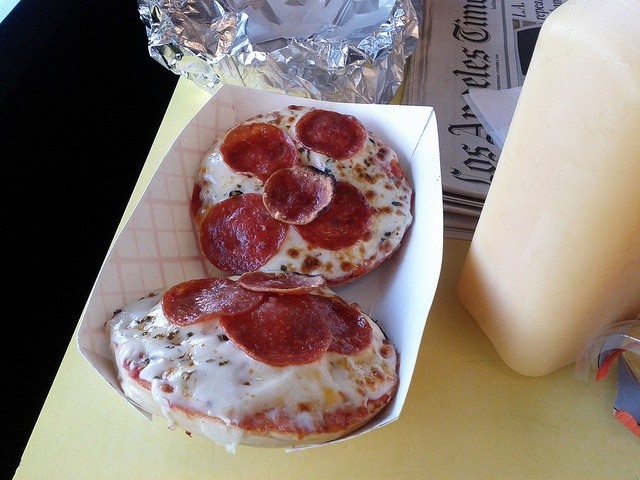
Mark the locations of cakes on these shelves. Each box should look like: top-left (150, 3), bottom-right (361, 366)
top-left (103, 272), bottom-right (398, 448)
top-left (190, 105), bottom-right (412, 285)
